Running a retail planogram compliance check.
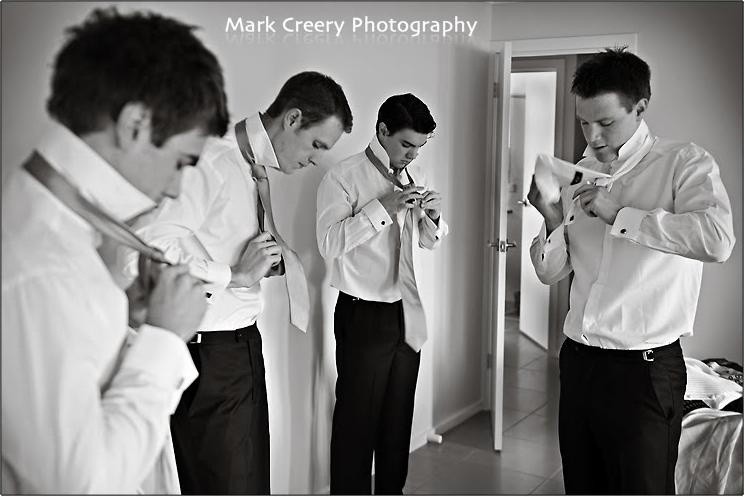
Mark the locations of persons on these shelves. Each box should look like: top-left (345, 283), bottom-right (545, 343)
top-left (525, 42), bottom-right (737, 496)
top-left (0, 1), bottom-right (233, 496)
top-left (313, 90), bottom-right (452, 495)
top-left (132, 67), bottom-right (356, 496)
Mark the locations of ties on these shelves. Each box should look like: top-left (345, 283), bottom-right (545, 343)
top-left (534, 133), bottom-right (658, 227)
top-left (233, 117), bottom-right (313, 334)
top-left (365, 144), bottom-right (432, 353)
top-left (21, 150), bottom-right (174, 298)
